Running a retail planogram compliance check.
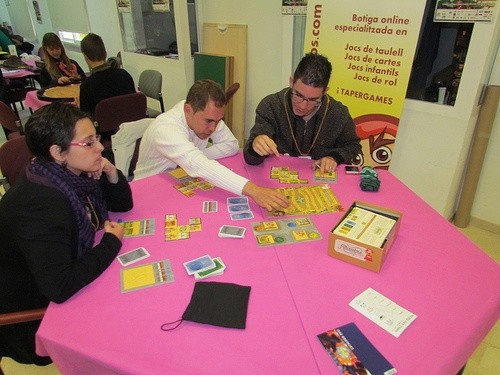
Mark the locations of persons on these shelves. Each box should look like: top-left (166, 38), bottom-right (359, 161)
top-left (38, 31), bottom-right (86, 89)
top-left (0, 21), bottom-right (34, 58)
top-left (78, 33), bottom-right (137, 115)
top-left (133, 79), bottom-right (291, 211)
top-left (243, 54), bottom-right (360, 177)
top-left (0, 101), bottom-right (134, 366)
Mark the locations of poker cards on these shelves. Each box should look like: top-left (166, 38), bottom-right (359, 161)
top-left (183, 254), bottom-right (227, 281)
top-left (116, 247), bottom-right (150, 267)
top-left (227, 196), bottom-right (254, 220)
top-left (218, 225), bottom-right (247, 239)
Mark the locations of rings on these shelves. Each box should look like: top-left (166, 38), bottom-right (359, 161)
top-left (329, 167), bottom-right (333, 169)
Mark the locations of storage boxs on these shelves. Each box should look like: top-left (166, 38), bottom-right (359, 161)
top-left (327, 199), bottom-right (402, 274)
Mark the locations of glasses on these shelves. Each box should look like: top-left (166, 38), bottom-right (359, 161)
top-left (291, 92), bottom-right (321, 105)
top-left (69, 135), bottom-right (101, 148)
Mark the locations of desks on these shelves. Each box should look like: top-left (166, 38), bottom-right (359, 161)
top-left (0, 59), bottom-right (40, 78)
top-left (35, 151), bottom-right (500, 375)
top-left (24, 81), bottom-right (81, 110)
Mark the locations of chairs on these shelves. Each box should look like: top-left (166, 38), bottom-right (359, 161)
top-left (0, 100), bottom-right (36, 186)
top-left (94, 69), bottom-right (165, 181)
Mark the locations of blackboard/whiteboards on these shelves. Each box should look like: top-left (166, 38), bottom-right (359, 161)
top-left (194, 53), bottom-right (234, 130)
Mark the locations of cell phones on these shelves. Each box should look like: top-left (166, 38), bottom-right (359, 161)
top-left (345, 165), bottom-right (359, 174)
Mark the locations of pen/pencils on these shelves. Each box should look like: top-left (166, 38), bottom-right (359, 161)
top-left (315, 163), bottom-right (320, 168)
